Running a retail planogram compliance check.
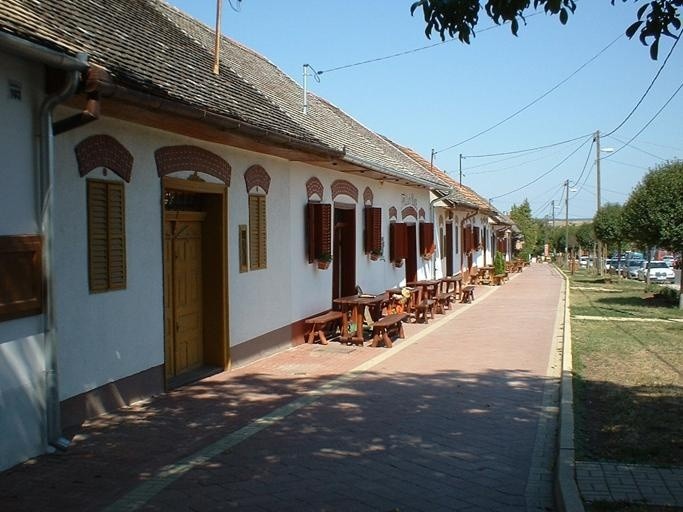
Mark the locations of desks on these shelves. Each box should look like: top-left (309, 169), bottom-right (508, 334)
top-left (440, 276), bottom-right (462, 299)
top-left (477, 267), bottom-right (495, 286)
top-left (408, 281), bottom-right (439, 307)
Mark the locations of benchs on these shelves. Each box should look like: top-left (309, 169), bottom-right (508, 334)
top-left (493, 272), bottom-right (507, 286)
top-left (306, 286), bottom-right (475, 349)
top-left (470, 273), bottom-right (478, 285)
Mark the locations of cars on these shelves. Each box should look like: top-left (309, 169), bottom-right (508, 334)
top-left (543, 248), bottom-right (682, 286)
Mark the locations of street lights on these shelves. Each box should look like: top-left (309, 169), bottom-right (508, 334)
top-left (565, 186), bottom-right (577, 269)
top-left (595, 146), bottom-right (614, 278)
top-left (551, 205), bottom-right (561, 261)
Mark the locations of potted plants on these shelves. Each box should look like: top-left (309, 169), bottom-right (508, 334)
top-left (317, 253), bottom-right (332, 270)
top-left (370, 248), bottom-right (380, 260)
